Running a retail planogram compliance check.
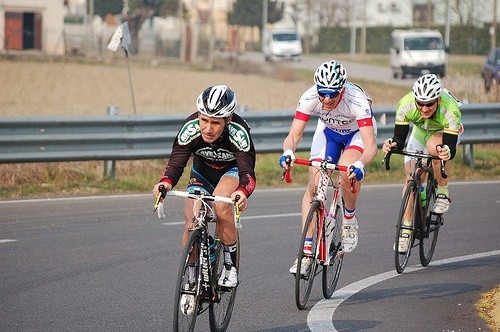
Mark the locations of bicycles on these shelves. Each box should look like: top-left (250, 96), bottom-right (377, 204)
top-left (281, 156), bottom-right (357, 310)
top-left (382, 142), bottom-right (447, 274)
top-left (153, 184), bottom-right (241, 332)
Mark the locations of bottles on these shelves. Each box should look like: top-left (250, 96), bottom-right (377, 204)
top-left (420, 185), bottom-right (427, 209)
top-left (208, 235), bottom-right (216, 262)
top-left (326, 209), bottom-right (336, 238)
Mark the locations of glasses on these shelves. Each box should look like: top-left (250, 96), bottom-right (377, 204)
top-left (317, 90), bottom-right (341, 99)
top-left (415, 99), bottom-right (437, 107)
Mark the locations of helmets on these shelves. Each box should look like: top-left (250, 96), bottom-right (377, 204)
top-left (412, 74), bottom-right (441, 102)
top-left (196, 85), bottom-right (238, 118)
top-left (314, 59), bottom-right (348, 89)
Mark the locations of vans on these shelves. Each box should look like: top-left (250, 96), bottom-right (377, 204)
top-left (263, 28), bottom-right (302, 62)
top-left (389, 29), bottom-right (449, 79)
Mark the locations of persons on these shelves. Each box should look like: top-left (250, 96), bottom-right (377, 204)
top-left (381, 73), bottom-right (464, 253)
top-left (153, 85), bottom-right (256, 315)
top-left (278, 60), bottom-right (379, 275)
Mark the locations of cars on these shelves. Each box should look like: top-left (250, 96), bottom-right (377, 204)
top-left (481, 48), bottom-right (500, 94)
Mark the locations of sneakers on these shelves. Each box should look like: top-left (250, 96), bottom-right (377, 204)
top-left (393, 230), bottom-right (413, 253)
top-left (218, 266), bottom-right (238, 287)
top-left (180, 283), bottom-right (197, 314)
top-left (341, 217), bottom-right (358, 253)
top-left (289, 257), bottom-right (313, 275)
top-left (431, 194), bottom-right (452, 214)
